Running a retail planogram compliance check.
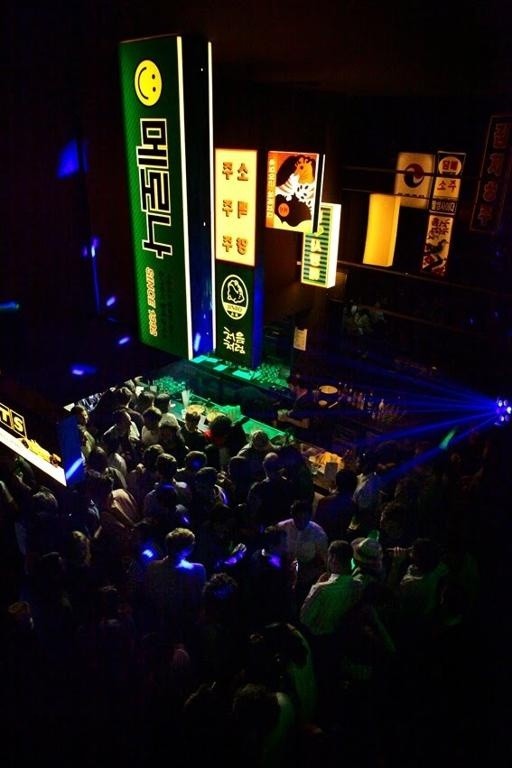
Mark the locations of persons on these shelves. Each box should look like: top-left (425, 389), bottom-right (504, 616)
top-left (1, 375), bottom-right (512, 767)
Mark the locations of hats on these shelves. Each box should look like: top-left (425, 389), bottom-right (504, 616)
top-left (351, 537), bottom-right (384, 569)
top-left (155, 453), bottom-right (177, 470)
